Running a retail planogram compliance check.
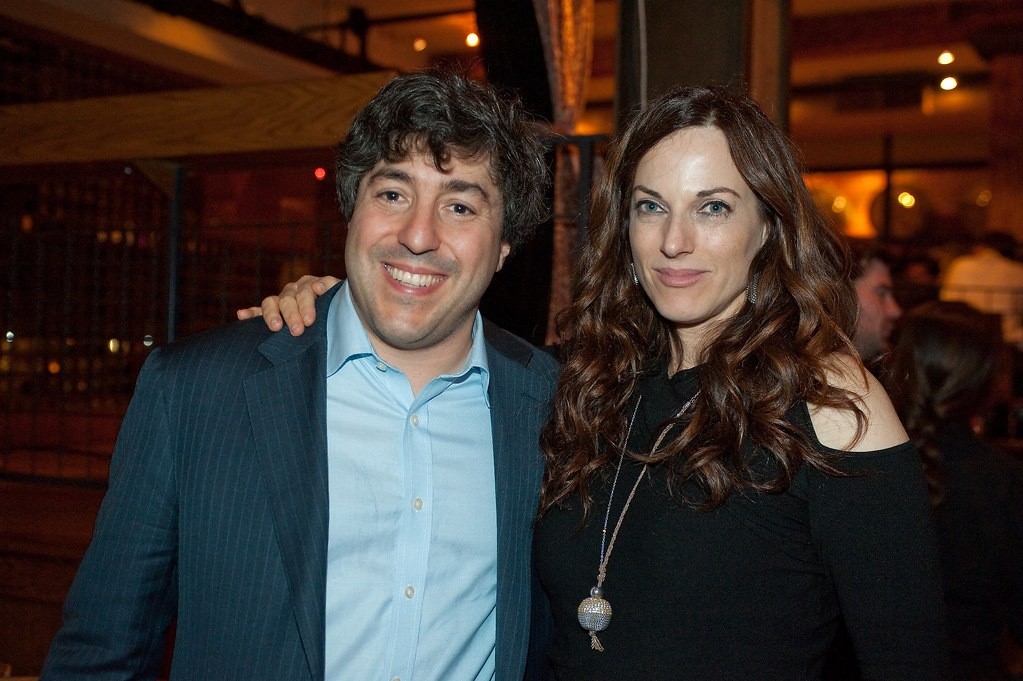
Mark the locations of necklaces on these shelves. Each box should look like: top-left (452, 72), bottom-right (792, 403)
top-left (577, 388), bottom-right (708, 651)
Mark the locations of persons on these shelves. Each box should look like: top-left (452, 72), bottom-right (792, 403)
top-left (38, 66), bottom-right (563, 681)
top-left (837, 226), bottom-right (1023, 681)
top-left (234, 81), bottom-right (944, 681)
